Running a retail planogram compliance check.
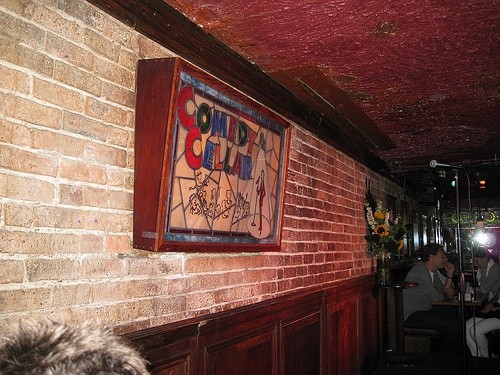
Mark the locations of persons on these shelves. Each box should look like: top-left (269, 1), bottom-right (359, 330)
top-left (403, 243), bottom-right (460, 354)
top-left (0, 316), bottom-right (151, 375)
top-left (466, 250), bottom-right (500, 357)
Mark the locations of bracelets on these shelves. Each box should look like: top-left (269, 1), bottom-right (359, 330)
top-left (446, 276), bottom-right (453, 279)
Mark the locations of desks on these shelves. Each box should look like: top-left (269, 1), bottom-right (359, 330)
top-left (431, 299), bottom-right (482, 316)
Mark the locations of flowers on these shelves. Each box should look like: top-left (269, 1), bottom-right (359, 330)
top-left (362, 188), bottom-right (417, 287)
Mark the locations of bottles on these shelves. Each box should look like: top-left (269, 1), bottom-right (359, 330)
top-left (465, 282), bottom-right (474, 298)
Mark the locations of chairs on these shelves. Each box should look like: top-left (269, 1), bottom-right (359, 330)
top-left (394, 267), bottom-right (443, 359)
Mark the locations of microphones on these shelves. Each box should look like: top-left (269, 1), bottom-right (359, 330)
top-left (429, 160), bottom-right (461, 169)
